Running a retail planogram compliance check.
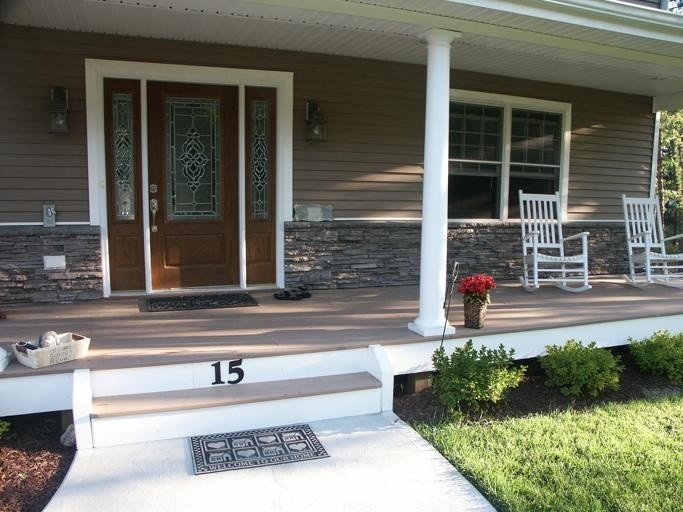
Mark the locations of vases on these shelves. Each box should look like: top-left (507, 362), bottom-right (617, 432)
top-left (464, 301), bottom-right (488, 329)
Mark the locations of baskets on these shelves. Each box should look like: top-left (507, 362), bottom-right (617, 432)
top-left (11, 332), bottom-right (91, 369)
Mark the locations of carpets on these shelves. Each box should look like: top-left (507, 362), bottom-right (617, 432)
top-left (189, 423), bottom-right (331, 476)
top-left (138, 292), bottom-right (261, 312)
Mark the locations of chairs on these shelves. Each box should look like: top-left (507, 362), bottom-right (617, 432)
top-left (518, 189), bottom-right (593, 293)
top-left (621, 194), bottom-right (683, 286)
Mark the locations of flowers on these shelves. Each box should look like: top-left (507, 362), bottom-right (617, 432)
top-left (457, 273), bottom-right (498, 305)
